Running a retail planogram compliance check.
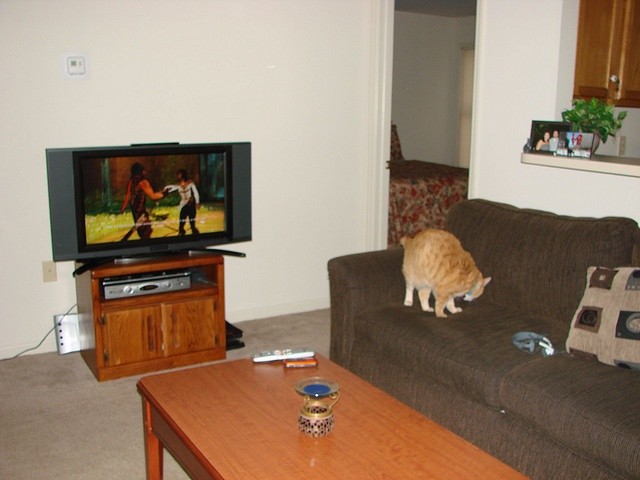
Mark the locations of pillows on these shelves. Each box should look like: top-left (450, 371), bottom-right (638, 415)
top-left (565, 266), bottom-right (640, 373)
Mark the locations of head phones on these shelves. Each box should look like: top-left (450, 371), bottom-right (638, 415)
top-left (510, 329), bottom-right (556, 359)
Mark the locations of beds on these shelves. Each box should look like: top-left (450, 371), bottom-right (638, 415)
top-left (388, 123), bottom-right (469, 249)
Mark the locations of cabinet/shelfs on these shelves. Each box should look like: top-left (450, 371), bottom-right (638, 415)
top-left (73, 247), bottom-right (228, 383)
top-left (572, 29), bottom-right (639, 109)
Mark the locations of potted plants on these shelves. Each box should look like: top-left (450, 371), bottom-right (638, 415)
top-left (561, 96), bottom-right (626, 154)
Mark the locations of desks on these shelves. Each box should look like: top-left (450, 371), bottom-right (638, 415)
top-left (137, 347), bottom-right (530, 480)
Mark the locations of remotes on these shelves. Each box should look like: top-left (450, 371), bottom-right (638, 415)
top-left (254, 348), bottom-right (315, 363)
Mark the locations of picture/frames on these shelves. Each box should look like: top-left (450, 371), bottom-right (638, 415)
top-left (556, 130), bottom-right (595, 158)
top-left (529, 118), bottom-right (573, 156)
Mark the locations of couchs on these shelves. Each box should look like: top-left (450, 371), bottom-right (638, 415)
top-left (327, 197), bottom-right (640, 480)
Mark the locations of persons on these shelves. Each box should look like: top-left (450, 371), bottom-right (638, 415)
top-left (163, 168), bottom-right (200, 236)
top-left (536, 132), bottom-right (550, 151)
top-left (119, 162), bottom-right (172, 242)
top-left (549, 131), bottom-right (560, 152)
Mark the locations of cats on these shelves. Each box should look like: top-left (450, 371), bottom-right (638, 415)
top-left (400, 229), bottom-right (492, 318)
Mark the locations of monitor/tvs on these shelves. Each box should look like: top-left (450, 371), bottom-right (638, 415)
top-left (45, 140), bottom-right (253, 264)
